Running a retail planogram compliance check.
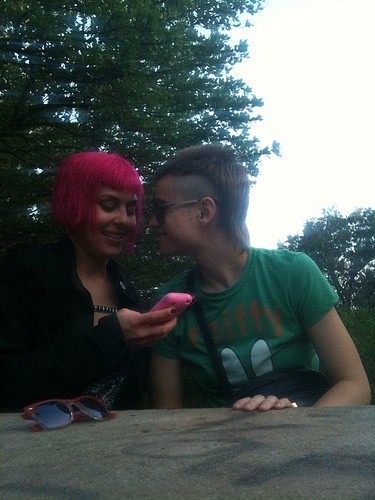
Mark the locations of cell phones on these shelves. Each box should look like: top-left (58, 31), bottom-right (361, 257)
top-left (147, 293), bottom-right (192, 326)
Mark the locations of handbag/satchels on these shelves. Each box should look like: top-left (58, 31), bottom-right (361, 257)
top-left (231, 367), bottom-right (329, 406)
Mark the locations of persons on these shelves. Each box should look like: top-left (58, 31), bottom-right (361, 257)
top-left (149, 145), bottom-right (371, 412)
top-left (1, 150), bottom-right (180, 412)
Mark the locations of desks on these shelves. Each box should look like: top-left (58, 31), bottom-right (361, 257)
top-left (0, 406), bottom-right (374, 500)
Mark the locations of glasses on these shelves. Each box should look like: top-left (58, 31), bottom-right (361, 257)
top-left (152, 199), bottom-right (199, 225)
top-left (23, 396), bottom-right (117, 432)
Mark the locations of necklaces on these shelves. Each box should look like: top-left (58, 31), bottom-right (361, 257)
top-left (92, 304), bottom-right (118, 314)
